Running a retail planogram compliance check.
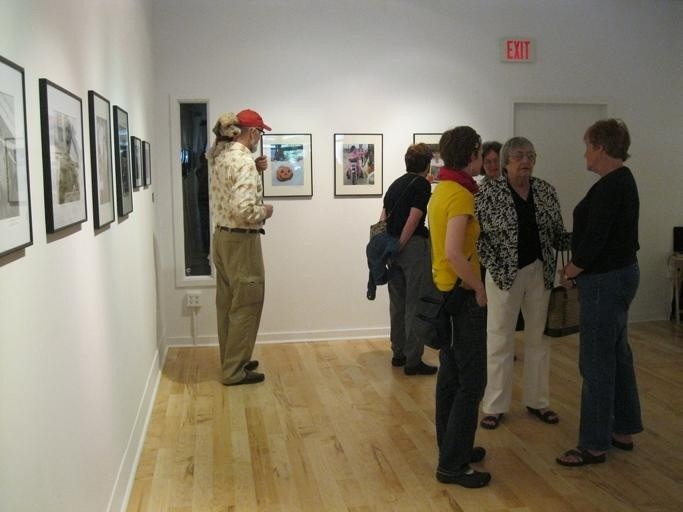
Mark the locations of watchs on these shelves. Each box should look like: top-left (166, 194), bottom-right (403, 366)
top-left (560, 266), bottom-right (574, 283)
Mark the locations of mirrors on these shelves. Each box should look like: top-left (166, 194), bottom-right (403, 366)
top-left (169, 94), bottom-right (217, 287)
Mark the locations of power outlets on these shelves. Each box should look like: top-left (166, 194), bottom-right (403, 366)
top-left (187, 293), bottom-right (201, 308)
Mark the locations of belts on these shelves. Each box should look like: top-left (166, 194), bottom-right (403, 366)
top-left (217, 225), bottom-right (265, 235)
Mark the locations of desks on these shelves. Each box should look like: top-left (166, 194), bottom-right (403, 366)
top-left (669, 253), bottom-right (683, 326)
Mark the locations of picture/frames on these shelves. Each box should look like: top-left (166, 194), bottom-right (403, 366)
top-left (130, 136), bottom-right (142, 188)
top-left (113, 105), bottom-right (133, 215)
top-left (0, 55), bottom-right (33, 256)
top-left (143, 141), bottom-right (151, 188)
top-left (38, 77), bottom-right (88, 233)
top-left (334, 133), bottom-right (384, 197)
top-left (414, 133), bottom-right (443, 195)
top-left (260, 133), bottom-right (313, 197)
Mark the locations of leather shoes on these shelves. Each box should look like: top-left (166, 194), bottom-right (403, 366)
top-left (404, 361), bottom-right (438, 375)
top-left (391, 356), bottom-right (407, 367)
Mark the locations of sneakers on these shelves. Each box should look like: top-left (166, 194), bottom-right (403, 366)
top-left (436, 468), bottom-right (491, 488)
top-left (223, 369), bottom-right (265, 385)
top-left (244, 360), bottom-right (259, 370)
top-left (469, 446), bottom-right (486, 463)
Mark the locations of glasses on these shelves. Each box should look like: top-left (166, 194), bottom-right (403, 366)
top-left (509, 154), bottom-right (536, 161)
top-left (256, 127), bottom-right (266, 136)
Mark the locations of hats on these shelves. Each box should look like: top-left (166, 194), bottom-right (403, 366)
top-left (234, 109), bottom-right (272, 131)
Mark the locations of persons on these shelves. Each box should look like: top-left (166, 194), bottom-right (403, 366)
top-left (474, 137), bottom-right (573, 429)
top-left (211, 108), bottom-right (275, 386)
top-left (556, 118), bottom-right (644, 466)
top-left (478, 140), bottom-right (504, 186)
top-left (375, 143), bottom-right (439, 376)
top-left (428, 126), bottom-right (492, 488)
top-left (345, 144), bottom-right (374, 184)
top-left (431, 149), bottom-right (445, 179)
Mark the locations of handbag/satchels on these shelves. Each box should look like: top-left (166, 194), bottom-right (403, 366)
top-left (370, 175), bottom-right (421, 239)
top-left (411, 254), bottom-right (472, 350)
top-left (543, 234), bottom-right (580, 338)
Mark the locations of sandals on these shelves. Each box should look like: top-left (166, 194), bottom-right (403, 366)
top-left (526, 406), bottom-right (559, 424)
top-left (480, 412), bottom-right (504, 429)
top-left (610, 436), bottom-right (634, 450)
top-left (556, 446), bottom-right (606, 466)
